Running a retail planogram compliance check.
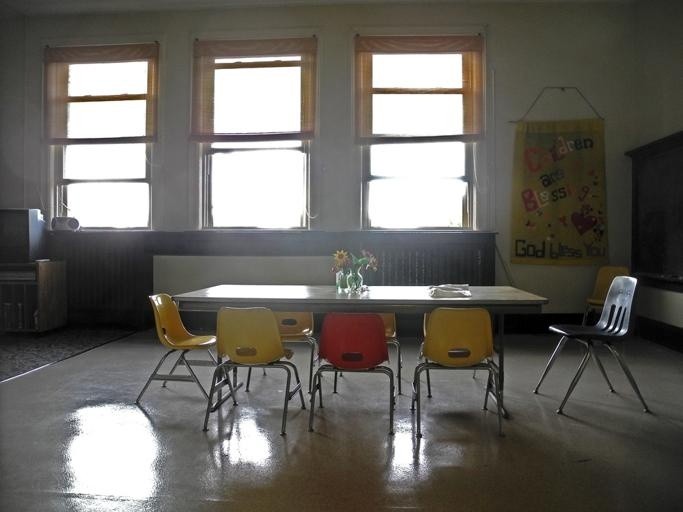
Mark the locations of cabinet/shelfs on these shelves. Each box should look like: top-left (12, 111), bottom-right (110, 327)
top-left (0, 261), bottom-right (68, 334)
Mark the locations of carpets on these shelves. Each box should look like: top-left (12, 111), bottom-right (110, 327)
top-left (0, 325), bottom-right (150, 384)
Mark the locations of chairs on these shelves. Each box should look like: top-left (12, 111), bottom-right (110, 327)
top-left (307, 311), bottom-right (397, 438)
top-left (332, 311), bottom-right (404, 396)
top-left (582, 266), bottom-right (631, 327)
top-left (244, 312), bottom-right (320, 394)
top-left (410, 306), bottom-right (505, 439)
top-left (531, 276), bottom-right (652, 413)
top-left (134, 292), bottom-right (227, 409)
top-left (420, 312), bottom-right (493, 396)
top-left (200, 305), bottom-right (305, 437)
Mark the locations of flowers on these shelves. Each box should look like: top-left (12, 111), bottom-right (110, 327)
top-left (342, 250), bottom-right (377, 288)
top-left (332, 249), bottom-right (349, 288)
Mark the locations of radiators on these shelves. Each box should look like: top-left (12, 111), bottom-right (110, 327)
top-left (151, 254), bottom-right (333, 313)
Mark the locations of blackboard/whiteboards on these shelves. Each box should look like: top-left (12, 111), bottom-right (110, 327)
top-left (624, 129), bottom-right (683, 294)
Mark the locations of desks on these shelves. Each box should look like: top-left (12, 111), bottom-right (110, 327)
top-left (169, 284), bottom-right (550, 416)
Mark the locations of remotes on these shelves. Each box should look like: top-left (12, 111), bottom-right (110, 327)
top-left (35, 259), bottom-right (50, 262)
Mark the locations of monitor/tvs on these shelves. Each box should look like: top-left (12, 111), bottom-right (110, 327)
top-left (0, 208), bottom-right (47, 263)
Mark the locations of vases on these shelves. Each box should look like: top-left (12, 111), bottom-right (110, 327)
top-left (346, 267), bottom-right (364, 296)
top-left (335, 266), bottom-right (351, 296)
